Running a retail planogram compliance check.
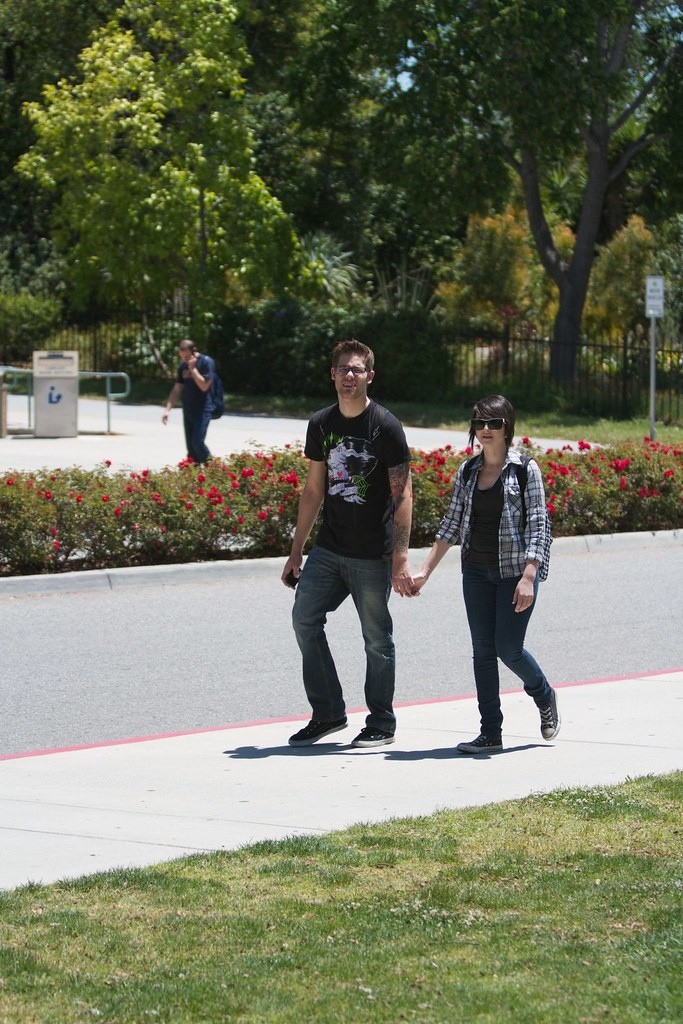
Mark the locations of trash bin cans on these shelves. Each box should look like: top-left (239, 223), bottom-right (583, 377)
top-left (32, 349), bottom-right (79, 437)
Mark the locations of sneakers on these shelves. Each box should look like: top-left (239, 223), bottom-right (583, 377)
top-left (457, 732), bottom-right (503, 754)
top-left (351, 727), bottom-right (394, 748)
top-left (289, 716), bottom-right (348, 746)
top-left (539, 687), bottom-right (561, 741)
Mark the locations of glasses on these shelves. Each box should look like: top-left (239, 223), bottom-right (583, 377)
top-left (469, 418), bottom-right (507, 430)
top-left (335, 366), bottom-right (369, 373)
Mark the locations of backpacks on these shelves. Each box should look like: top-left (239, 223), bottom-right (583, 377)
top-left (183, 355), bottom-right (224, 419)
top-left (463, 454), bottom-right (553, 582)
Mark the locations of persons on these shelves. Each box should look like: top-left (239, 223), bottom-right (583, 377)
top-left (162, 339), bottom-right (215, 468)
top-left (410, 395), bottom-right (562, 754)
top-left (281, 338), bottom-right (416, 748)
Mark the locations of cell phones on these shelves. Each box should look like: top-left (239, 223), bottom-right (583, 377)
top-left (286, 568), bottom-right (302, 587)
top-left (192, 347), bottom-right (197, 356)
